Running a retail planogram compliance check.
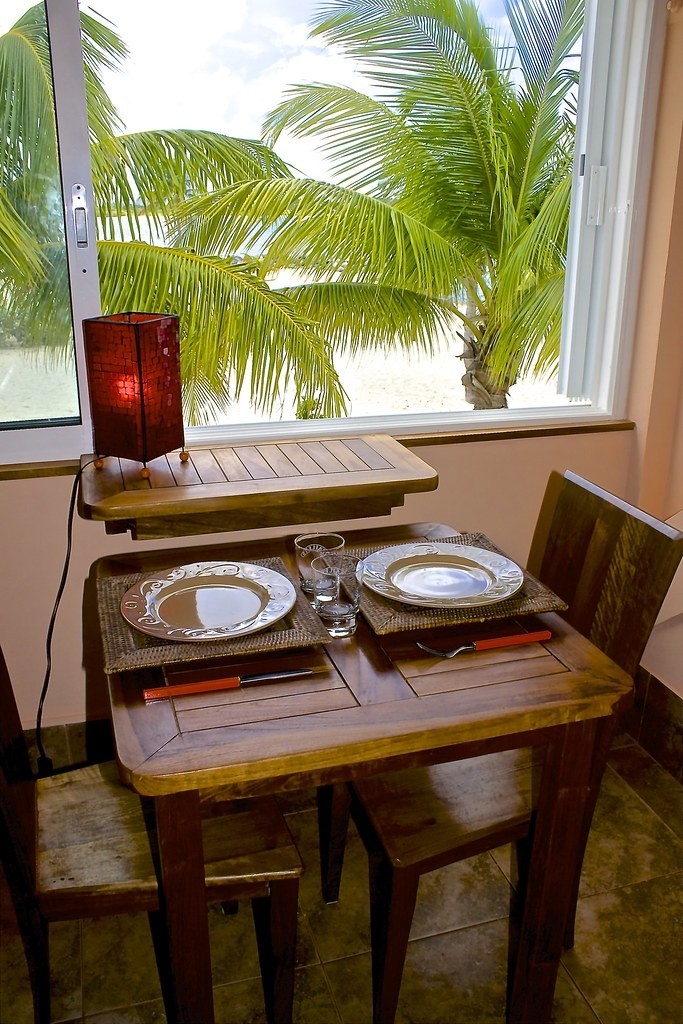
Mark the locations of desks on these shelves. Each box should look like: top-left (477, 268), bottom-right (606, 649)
top-left (77, 432), bottom-right (438, 542)
top-left (82, 519), bottom-right (639, 1024)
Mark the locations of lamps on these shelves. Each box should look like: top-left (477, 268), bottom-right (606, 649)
top-left (82, 312), bottom-right (190, 479)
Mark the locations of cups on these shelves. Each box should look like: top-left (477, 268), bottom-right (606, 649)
top-left (311, 555), bottom-right (365, 637)
top-left (295, 532), bottom-right (345, 593)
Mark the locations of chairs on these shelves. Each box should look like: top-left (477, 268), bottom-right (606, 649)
top-left (0, 647), bottom-right (305, 1024)
top-left (312, 469), bottom-right (683, 1024)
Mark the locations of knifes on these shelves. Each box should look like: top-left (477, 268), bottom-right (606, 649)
top-left (143, 670), bottom-right (313, 699)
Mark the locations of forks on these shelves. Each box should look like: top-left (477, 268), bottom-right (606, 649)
top-left (416, 630), bottom-right (551, 657)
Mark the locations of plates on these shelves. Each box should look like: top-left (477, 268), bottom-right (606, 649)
top-left (121, 561), bottom-right (296, 641)
top-left (357, 544), bottom-right (523, 608)
